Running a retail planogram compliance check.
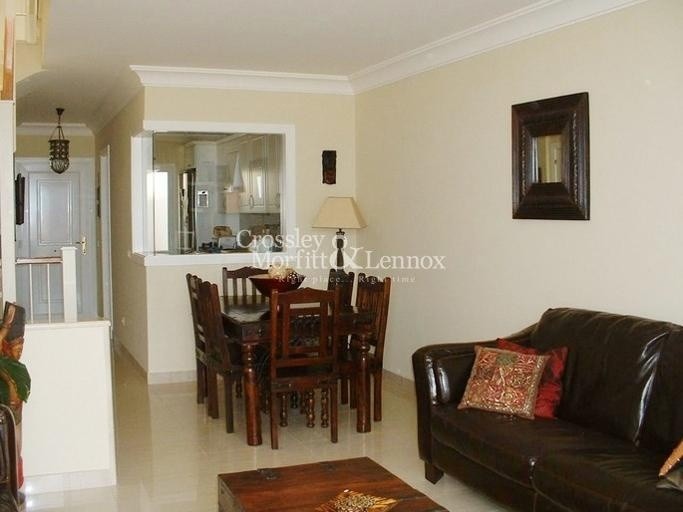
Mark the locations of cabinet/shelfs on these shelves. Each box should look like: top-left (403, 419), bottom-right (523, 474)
top-left (216, 133), bottom-right (280, 214)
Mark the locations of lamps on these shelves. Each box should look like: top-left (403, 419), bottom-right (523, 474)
top-left (311, 198), bottom-right (368, 270)
top-left (48, 109), bottom-right (69, 173)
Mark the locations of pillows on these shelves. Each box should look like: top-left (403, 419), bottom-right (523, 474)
top-left (457, 346), bottom-right (550, 420)
top-left (656, 441), bottom-right (683, 491)
top-left (496, 338), bottom-right (568, 420)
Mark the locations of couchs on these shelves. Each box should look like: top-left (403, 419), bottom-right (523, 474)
top-left (413, 308), bottom-right (683, 512)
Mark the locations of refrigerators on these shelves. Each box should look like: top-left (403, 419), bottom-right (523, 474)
top-left (178, 166), bottom-right (195, 249)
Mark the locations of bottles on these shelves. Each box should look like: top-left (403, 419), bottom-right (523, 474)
top-left (273, 226), bottom-right (280, 251)
top-left (262, 226), bottom-right (272, 252)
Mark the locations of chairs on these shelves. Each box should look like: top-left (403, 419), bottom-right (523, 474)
top-left (186, 267), bottom-right (391, 449)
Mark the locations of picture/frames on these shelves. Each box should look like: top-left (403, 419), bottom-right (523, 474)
top-left (511, 91), bottom-right (590, 220)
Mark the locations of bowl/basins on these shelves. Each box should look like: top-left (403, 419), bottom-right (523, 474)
top-left (248, 272), bottom-right (305, 298)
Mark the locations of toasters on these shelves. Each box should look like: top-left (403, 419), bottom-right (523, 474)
top-left (216, 234), bottom-right (238, 250)
